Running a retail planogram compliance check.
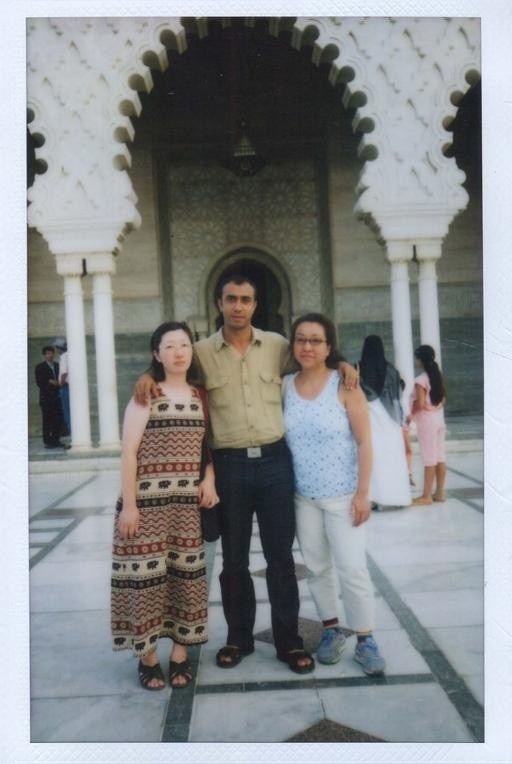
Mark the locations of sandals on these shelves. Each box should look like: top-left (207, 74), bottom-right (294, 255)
top-left (217, 646), bottom-right (255, 667)
top-left (432, 494), bottom-right (446, 502)
top-left (277, 650), bottom-right (316, 674)
top-left (170, 658), bottom-right (194, 688)
top-left (414, 497), bottom-right (431, 505)
top-left (139, 660), bottom-right (165, 690)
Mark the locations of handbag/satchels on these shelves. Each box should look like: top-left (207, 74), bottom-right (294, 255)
top-left (201, 507), bottom-right (220, 542)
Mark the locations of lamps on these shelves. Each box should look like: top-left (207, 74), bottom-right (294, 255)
top-left (232, 120), bottom-right (257, 171)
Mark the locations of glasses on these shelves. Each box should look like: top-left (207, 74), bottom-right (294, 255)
top-left (296, 334), bottom-right (325, 345)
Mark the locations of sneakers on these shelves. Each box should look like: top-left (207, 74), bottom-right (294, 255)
top-left (318, 630), bottom-right (346, 663)
top-left (354, 639), bottom-right (384, 675)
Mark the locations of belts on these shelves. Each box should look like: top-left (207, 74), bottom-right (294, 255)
top-left (220, 444), bottom-right (280, 459)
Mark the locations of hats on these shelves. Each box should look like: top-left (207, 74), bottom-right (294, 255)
top-left (52, 338), bottom-right (67, 349)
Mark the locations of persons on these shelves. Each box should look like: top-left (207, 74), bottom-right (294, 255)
top-left (354, 332), bottom-right (451, 512)
top-left (277, 308), bottom-right (383, 678)
top-left (33, 336), bottom-right (74, 451)
top-left (110, 321), bottom-right (223, 689)
top-left (131, 268), bottom-right (361, 673)
